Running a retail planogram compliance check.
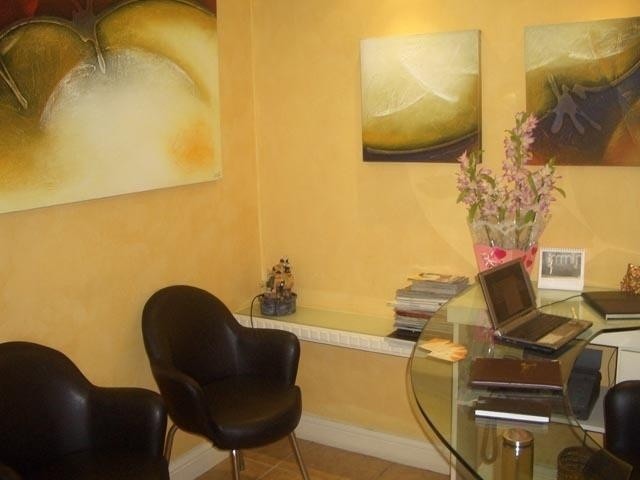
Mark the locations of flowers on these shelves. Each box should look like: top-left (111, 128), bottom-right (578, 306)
top-left (454, 110), bottom-right (566, 248)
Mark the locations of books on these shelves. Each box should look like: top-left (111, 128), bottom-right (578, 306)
top-left (468, 357), bottom-right (563, 390)
top-left (474, 396), bottom-right (551, 423)
top-left (393, 271), bottom-right (469, 332)
top-left (581, 291), bottom-right (640, 320)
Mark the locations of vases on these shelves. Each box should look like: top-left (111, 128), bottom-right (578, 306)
top-left (467, 216), bottom-right (543, 273)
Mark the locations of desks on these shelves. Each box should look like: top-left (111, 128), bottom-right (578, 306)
top-left (233, 305), bottom-right (451, 473)
top-left (406, 268), bottom-right (640, 480)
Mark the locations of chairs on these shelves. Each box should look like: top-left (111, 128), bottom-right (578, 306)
top-left (141, 284), bottom-right (310, 480)
top-left (1, 341), bottom-right (170, 480)
top-left (604, 380), bottom-right (639, 480)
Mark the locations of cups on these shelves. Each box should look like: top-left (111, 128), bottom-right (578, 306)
top-left (501, 430), bottom-right (532, 479)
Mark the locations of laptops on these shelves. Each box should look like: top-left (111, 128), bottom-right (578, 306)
top-left (477, 257), bottom-right (594, 354)
